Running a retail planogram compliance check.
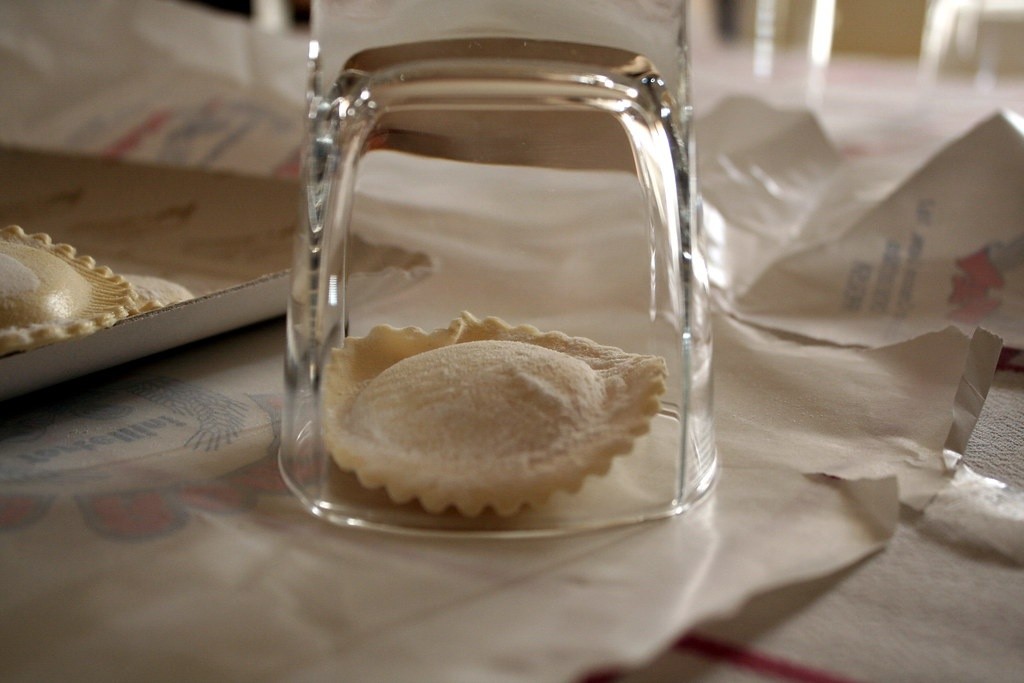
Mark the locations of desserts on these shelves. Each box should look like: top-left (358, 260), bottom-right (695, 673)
top-left (0, 222), bottom-right (136, 355)
top-left (319, 310), bottom-right (669, 520)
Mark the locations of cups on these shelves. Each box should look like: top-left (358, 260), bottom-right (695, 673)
top-left (272, 42), bottom-right (718, 537)
